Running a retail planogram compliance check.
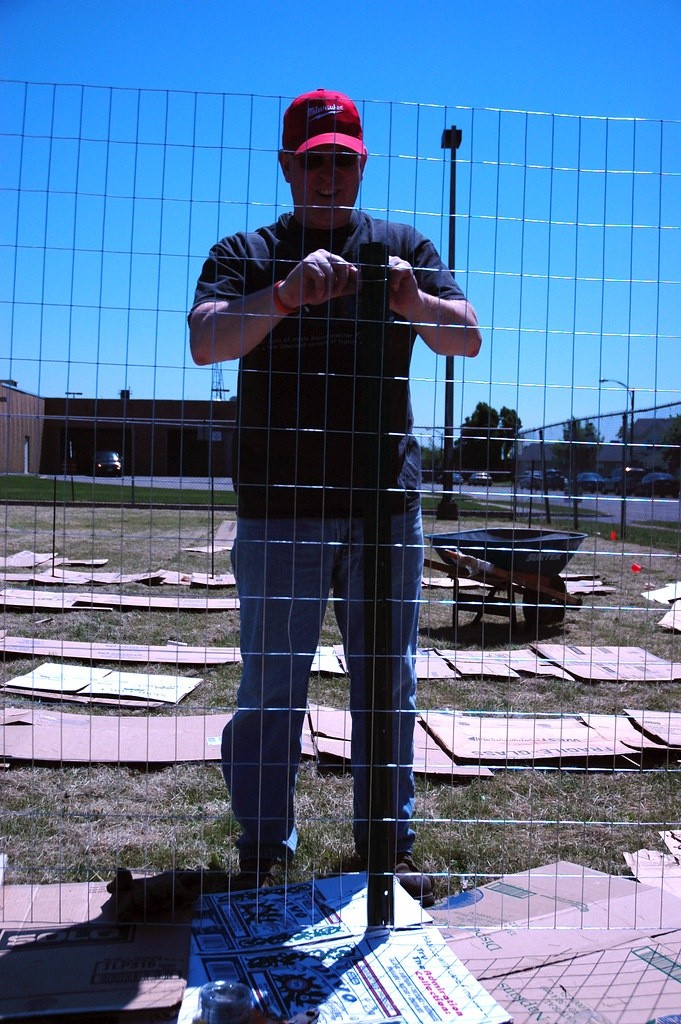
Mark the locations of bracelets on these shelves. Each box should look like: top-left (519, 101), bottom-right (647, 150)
top-left (273, 280), bottom-right (298, 314)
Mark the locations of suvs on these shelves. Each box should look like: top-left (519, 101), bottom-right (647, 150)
top-left (601, 467), bottom-right (648, 496)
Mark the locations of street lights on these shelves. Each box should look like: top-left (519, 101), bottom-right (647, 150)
top-left (433, 125), bottom-right (462, 519)
top-left (599, 377), bottom-right (634, 462)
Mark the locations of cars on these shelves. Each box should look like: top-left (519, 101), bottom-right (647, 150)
top-left (571, 472), bottom-right (609, 492)
top-left (636, 472), bottom-right (681, 499)
top-left (92, 450), bottom-right (122, 477)
top-left (545, 468), bottom-right (565, 492)
top-left (519, 473), bottom-right (544, 489)
top-left (437, 474), bottom-right (465, 485)
top-left (468, 472), bottom-right (493, 487)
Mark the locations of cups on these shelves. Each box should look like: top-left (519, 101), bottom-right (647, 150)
top-left (199, 981), bottom-right (251, 1024)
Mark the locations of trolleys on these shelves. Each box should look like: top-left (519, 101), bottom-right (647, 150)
top-left (424, 526), bottom-right (589, 626)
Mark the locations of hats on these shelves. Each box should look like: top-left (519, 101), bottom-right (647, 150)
top-left (279, 90), bottom-right (365, 154)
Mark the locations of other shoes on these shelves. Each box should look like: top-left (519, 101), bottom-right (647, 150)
top-left (367, 853), bottom-right (435, 898)
top-left (215, 865), bottom-right (274, 892)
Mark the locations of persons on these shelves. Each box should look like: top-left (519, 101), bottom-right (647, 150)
top-left (189, 89), bottom-right (481, 907)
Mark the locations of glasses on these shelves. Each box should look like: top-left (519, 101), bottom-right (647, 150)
top-left (293, 151), bottom-right (361, 170)
